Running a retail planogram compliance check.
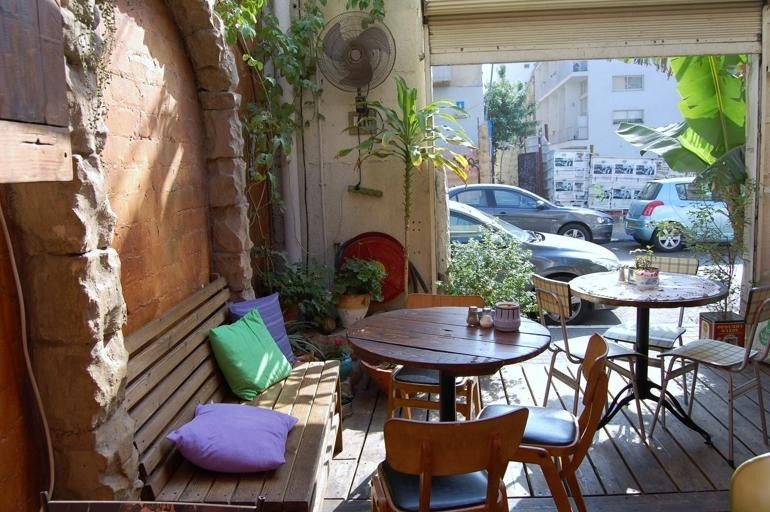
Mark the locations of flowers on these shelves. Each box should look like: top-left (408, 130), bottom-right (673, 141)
top-left (324, 336), bottom-right (345, 358)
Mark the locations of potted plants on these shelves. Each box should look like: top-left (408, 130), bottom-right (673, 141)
top-left (251, 241), bottom-right (331, 336)
top-left (641, 160), bottom-right (767, 351)
top-left (634, 240), bottom-right (661, 292)
top-left (329, 255), bottom-right (388, 331)
top-left (359, 352), bottom-right (419, 402)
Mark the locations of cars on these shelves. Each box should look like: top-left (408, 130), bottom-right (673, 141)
top-left (448, 182), bottom-right (616, 248)
top-left (624, 176), bottom-right (737, 251)
top-left (445, 201), bottom-right (625, 324)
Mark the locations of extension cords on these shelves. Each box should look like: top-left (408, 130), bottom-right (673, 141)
top-left (347, 185), bottom-right (382, 197)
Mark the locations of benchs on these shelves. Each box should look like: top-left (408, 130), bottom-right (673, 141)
top-left (115, 268), bottom-right (346, 512)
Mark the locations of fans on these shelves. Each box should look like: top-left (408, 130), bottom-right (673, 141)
top-left (315, 10), bottom-right (397, 138)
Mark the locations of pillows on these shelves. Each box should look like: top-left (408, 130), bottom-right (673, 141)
top-left (167, 400), bottom-right (299, 475)
top-left (206, 306), bottom-right (292, 401)
top-left (225, 291), bottom-right (303, 370)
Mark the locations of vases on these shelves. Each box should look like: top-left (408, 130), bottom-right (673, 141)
top-left (323, 350), bottom-right (354, 380)
top-left (340, 398), bottom-right (355, 421)
top-left (339, 374), bottom-right (356, 405)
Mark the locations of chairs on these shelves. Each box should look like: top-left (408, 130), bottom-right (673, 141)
top-left (530, 271), bottom-right (651, 446)
top-left (367, 405), bottom-right (532, 511)
top-left (727, 451), bottom-right (770, 512)
top-left (384, 291), bottom-right (487, 422)
top-left (598, 253), bottom-right (701, 430)
top-left (473, 331), bottom-right (609, 511)
top-left (646, 282), bottom-right (770, 469)
